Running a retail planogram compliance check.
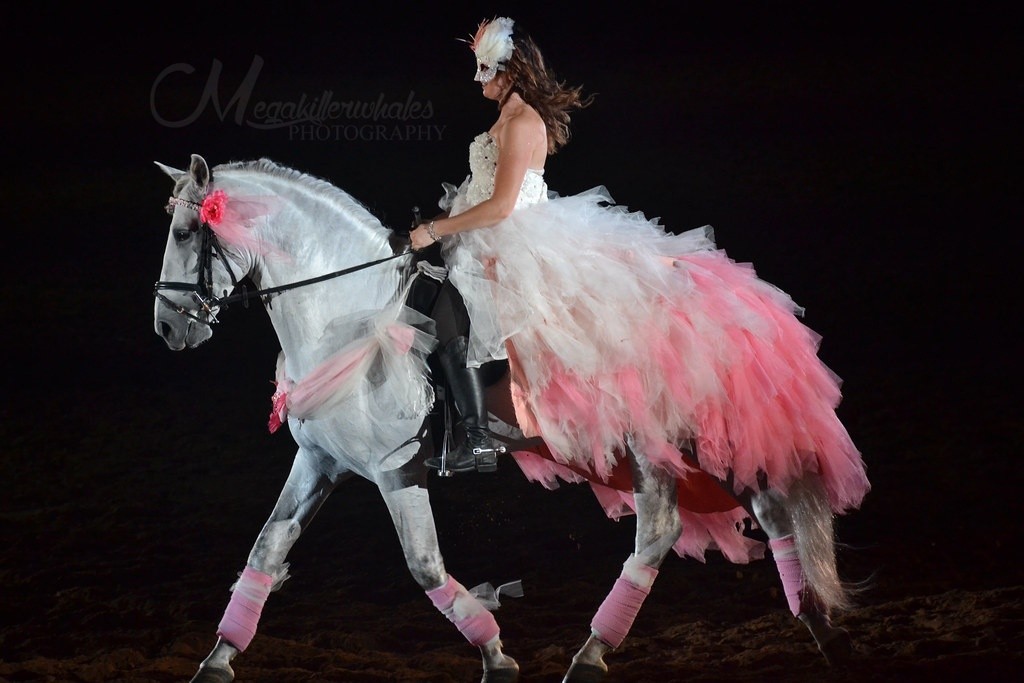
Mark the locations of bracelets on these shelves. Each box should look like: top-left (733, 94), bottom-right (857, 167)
top-left (428, 221), bottom-right (442, 240)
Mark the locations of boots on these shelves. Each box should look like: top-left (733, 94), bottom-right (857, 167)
top-left (424, 335), bottom-right (498, 474)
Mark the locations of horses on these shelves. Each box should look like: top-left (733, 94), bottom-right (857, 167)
top-left (147, 151), bottom-right (894, 683)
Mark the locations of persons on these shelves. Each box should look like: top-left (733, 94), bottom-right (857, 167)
top-left (411, 18), bottom-right (582, 472)
